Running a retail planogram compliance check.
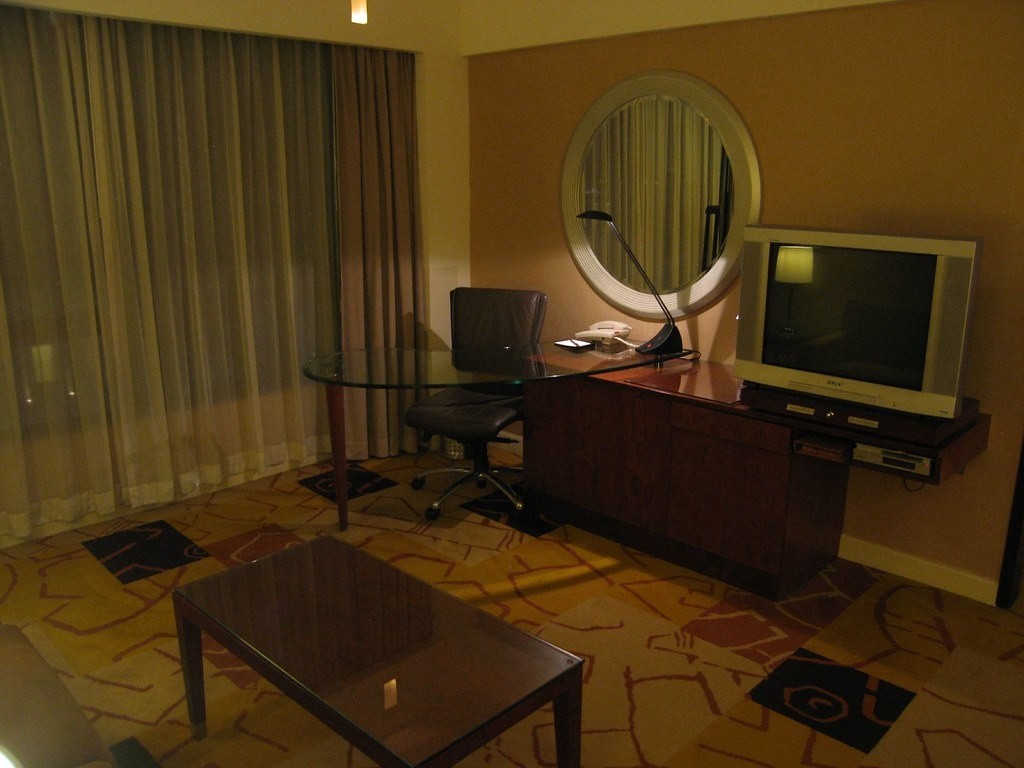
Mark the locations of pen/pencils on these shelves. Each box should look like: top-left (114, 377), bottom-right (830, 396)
top-left (570, 338), bottom-right (579, 348)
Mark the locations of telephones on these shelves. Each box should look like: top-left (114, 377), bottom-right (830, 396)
top-left (575, 320), bottom-right (631, 345)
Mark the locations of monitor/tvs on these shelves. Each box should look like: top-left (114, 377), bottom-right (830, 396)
top-left (734, 224), bottom-right (983, 418)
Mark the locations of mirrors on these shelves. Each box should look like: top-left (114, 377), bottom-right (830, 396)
top-left (559, 70), bottom-right (764, 322)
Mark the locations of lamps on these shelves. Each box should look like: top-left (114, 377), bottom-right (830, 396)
top-left (576, 210), bottom-right (683, 355)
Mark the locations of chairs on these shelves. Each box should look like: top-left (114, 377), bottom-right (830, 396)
top-left (404, 287), bottom-right (547, 523)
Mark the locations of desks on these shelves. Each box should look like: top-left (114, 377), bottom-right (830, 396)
top-left (173, 536), bottom-right (586, 768)
top-left (302, 348), bottom-right (691, 531)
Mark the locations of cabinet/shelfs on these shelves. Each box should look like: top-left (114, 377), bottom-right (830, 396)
top-left (523, 342), bottom-right (991, 601)
